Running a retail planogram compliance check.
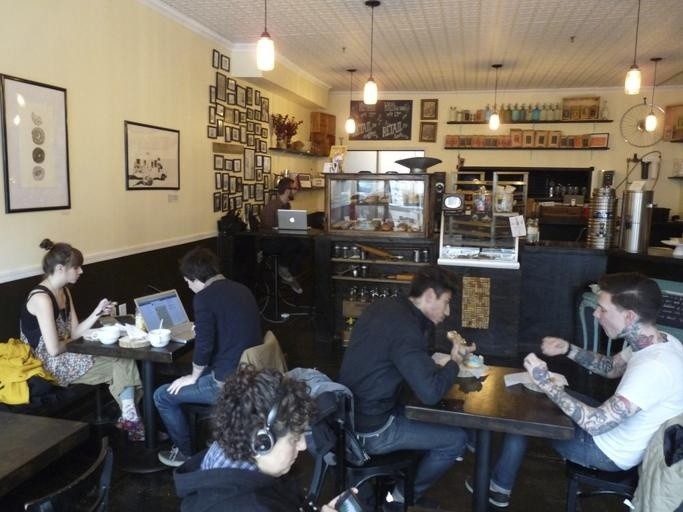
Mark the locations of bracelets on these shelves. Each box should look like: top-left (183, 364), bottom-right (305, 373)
top-left (561, 342), bottom-right (571, 357)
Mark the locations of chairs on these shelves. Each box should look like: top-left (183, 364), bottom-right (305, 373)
top-left (566, 422), bottom-right (683, 512)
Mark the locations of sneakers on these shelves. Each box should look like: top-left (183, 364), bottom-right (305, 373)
top-left (158, 448), bottom-right (192, 467)
top-left (277, 267), bottom-right (305, 295)
top-left (127, 428), bottom-right (169, 442)
top-left (384, 493), bottom-right (440, 512)
top-left (464, 476), bottom-right (511, 507)
top-left (115, 415), bottom-right (162, 438)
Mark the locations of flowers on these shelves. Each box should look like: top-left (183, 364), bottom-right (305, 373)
top-left (271, 113), bottom-right (303, 139)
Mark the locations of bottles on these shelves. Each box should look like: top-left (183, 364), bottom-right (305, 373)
top-left (601, 100), bottom-right (609, 120)
top-left (526, 218), bottom-right (540, 244)
top-left (547, 182), bottom-right (590, 217)
top-left (334, 243), bottom-right (430, 330)
top-left (449, 103), bottom-right (562, 121)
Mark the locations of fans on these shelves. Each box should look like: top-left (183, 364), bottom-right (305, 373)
top-left (620, 97), bottom-right (666, 147)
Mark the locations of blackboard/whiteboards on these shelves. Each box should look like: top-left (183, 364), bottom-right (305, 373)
top-left (455, 168), bottom-right (592, 203)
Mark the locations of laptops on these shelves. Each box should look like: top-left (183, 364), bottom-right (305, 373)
top-left (133, 287), bottom-right (197, 346)
top-left (277, 208), bottom-right (313, 230)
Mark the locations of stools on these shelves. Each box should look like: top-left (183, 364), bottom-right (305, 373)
top-left (578, 292), bottom-right (628, 376)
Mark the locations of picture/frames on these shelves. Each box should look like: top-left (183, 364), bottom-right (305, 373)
top-left (445, 128), bottom-right (610, 148)
top-left (419, 122), bottom-right (438, 143)
top-left (124, 120), bottom-right (180, 190)
top-left (0, 73), bottom-right (71, 213)
top-left (207, 48), bottom-right (272, 221)
top-left (420, 99), bottom-right (438, 120)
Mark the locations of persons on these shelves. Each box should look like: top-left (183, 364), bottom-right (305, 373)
top-left (260, 177), bottom-right (313, 296)
top-left (463, 271), bottom-right (683, 509)
top-left (151, 246), bottom-right (261, 468)
top-left (172, 361), bottom-right (359, 512)
top-left (19, 237), bottom-right (170, 444)
top-left (338, 264), bottom-right (469, 512)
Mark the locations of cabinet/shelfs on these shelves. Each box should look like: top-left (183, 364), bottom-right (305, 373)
top-left (449, 171), bottom-right (529, 249)
top-left (445, 119), bottom-right (613, 150)
top-left (333, 246), bottom-right (428, 284)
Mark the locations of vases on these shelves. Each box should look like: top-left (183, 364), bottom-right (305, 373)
top-left (277, 137), bottom-right (285, 147)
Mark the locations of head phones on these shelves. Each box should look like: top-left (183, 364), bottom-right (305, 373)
top-left (249, 377), bottom-right (291, 457)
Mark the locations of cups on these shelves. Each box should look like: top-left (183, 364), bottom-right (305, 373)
top-left (92, 326), bottom-right (120, 345)
top-left (149, 329), bottom-right (172, 348)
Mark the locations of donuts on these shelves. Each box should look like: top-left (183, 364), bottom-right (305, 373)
top-left (32, 127), bottom-right (45, 181)
